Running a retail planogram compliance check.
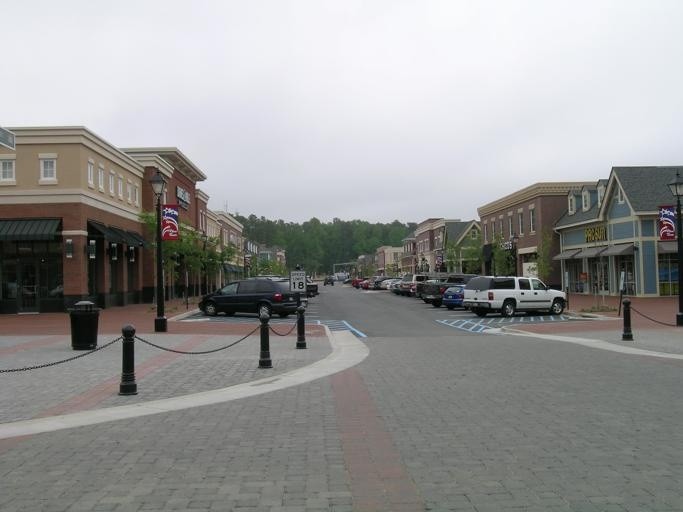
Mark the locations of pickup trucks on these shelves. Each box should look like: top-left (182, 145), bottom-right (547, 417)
top-left (461, 275), bottom-right (568, 318)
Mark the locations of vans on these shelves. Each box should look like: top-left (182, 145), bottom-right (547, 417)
top-left (195, 274), bottom-right (307, 319)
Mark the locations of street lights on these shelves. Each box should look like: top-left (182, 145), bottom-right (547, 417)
top-left (434, 249), bottom-right (444, 264)
top-left (510, 231), bottom-right (518, 277)
top-left (665, 165), bottom-right (682, 326)
top-left (148, 164), bottom-right (167, 333)
top-left (201, 230), bottom-right (209, 252)
top-left (242, 246), bottom-right (251, 277)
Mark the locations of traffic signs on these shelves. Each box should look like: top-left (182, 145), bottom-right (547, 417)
top-left (288, 270), bottom-right (307, 292)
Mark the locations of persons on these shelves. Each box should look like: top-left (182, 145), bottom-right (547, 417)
top-left (51, 280), bottom-right (63, 296)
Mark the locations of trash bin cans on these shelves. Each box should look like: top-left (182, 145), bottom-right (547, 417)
top-left (67, 301), bottom-right (102, 350)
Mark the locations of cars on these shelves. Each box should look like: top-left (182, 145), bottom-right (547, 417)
top-left (323, 269), bottom-right (478, 310)
top-left (254, 272), bottom-right (319, 299)
